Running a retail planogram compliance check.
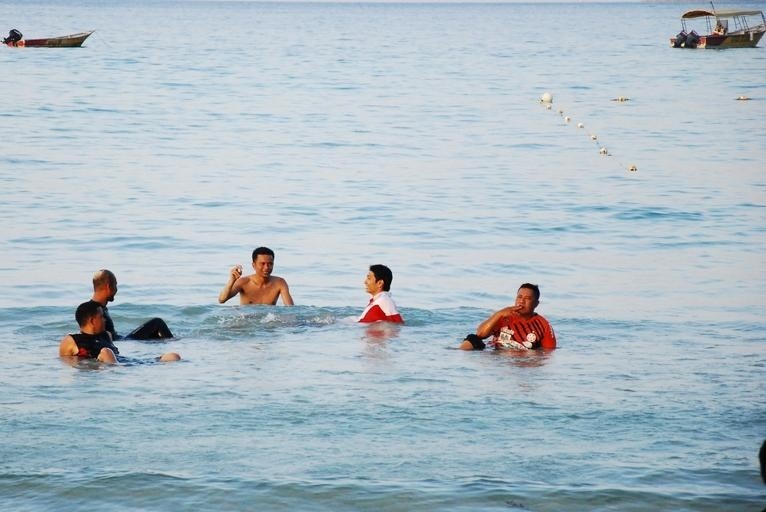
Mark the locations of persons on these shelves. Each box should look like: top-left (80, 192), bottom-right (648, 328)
top-left (60, 302), bottom-right (181, 364)
top-left (219, 247), bottom-right (294, 307)
top-left (458, 283), bottom-right (557, 358)
top-left (712, 22), bottom-right (727, 35)
top-left (356, 264), bottom-right (404, 337)
top-left (91, 270), bottom-right (173, 341)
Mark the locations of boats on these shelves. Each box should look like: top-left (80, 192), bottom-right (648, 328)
top-left (1, 26), bottom-right (94, 50)
top-left (669, 1), bottom-right (766, 50)
